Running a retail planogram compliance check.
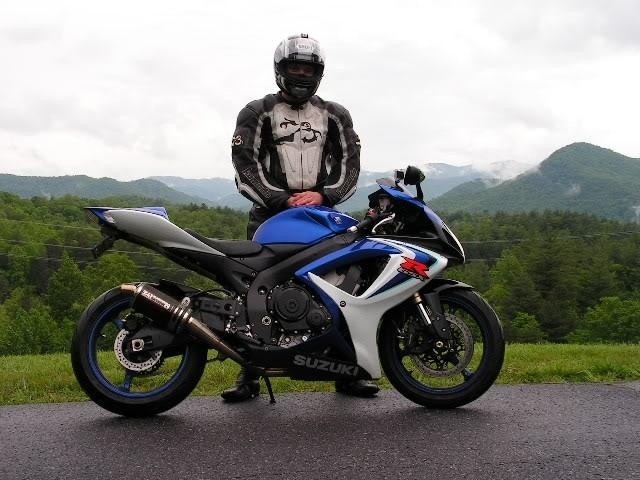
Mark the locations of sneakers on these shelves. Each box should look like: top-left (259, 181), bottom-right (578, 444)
top-left (221, 381), bottom-right (260, 400)
top-left (335, 380), bottom-right (380, 394)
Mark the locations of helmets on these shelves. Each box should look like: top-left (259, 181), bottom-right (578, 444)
top-left (274, 33), bottom-right (324, 99)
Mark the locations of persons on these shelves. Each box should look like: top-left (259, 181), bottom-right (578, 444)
top-left (221, 33), bottom-right (379, 400)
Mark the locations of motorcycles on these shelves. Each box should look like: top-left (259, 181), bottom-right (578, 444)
top-left (70, 164), bottom-right (505, 419)
